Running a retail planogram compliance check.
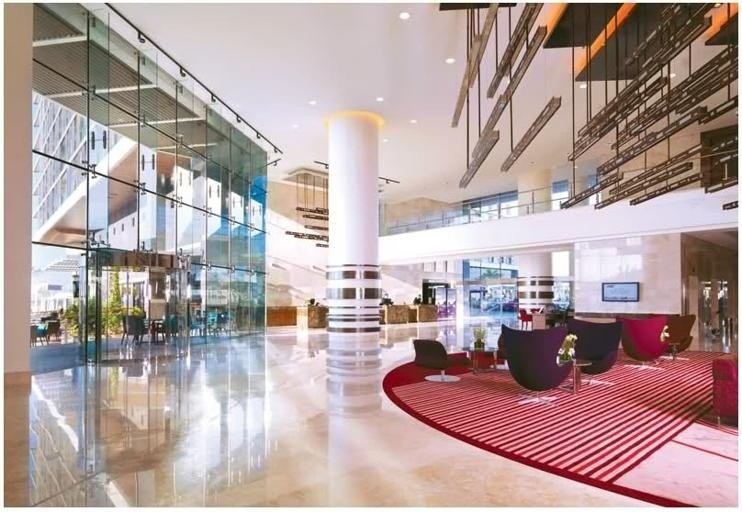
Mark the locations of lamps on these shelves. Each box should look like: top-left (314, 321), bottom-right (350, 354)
top-left (283, 169), bottom-right (329, 247)
top-left (561, 4), bottom-right (738, 211)
top-left (438, 2), bottom-right (561, 189)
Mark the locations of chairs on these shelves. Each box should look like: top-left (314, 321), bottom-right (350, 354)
top-left (120, 310), bottom-right (238, 349)
top-left (412, 339), bottom-right (471, 383)
top-left (31, 318), bottom-right (64, 346)
top-left (501, 313), bottom-right (696, 408)
top-left (519, 309), bottom-right (532, 330)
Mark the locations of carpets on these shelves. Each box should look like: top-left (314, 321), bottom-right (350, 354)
top-left (381, 351), bottom-right (738, 507)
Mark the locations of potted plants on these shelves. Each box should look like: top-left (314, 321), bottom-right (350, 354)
top-left (472, 327), bottom-right (488, 350)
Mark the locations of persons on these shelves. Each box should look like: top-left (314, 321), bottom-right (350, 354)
top-left (413, 294), bottom-right (422, 305)
top-left (424, 296), bottom-right (435, 305)
top-left (309, 298), bottom-right (320, 307)
